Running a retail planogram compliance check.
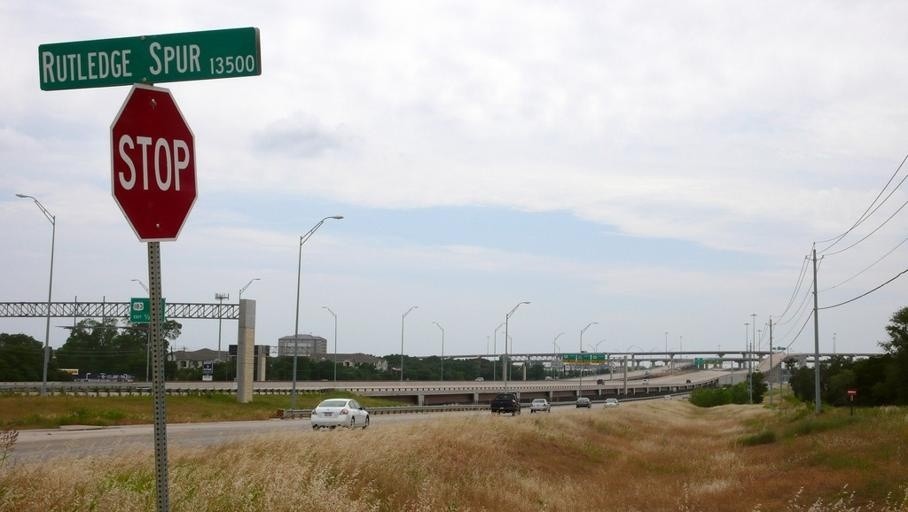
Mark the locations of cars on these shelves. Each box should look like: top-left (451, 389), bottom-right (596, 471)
top-left (530, 397), bottom-right (553, 415)
top-left (57, 366), bottom-right (135, 387)
top-left (663, 395), bottom-right (671, 400)
top-left (309, 397), bottom-right (370, 429)
top-left (601, 396), bottom-right (620, 407)
top-left (641, 378), bottom-right (650, 384)
top-left (595, 378), bottom-right (607, 386)
top-left (474, 377), bottom-right (484, 382)
top-left (574, 397), bottom-right (593, 408)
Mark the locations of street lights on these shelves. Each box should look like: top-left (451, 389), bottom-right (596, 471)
top-left (320, 302), bottom-right (339, 381)
top-left (429, 319), bottom-right (446, 382)
top-left (289, 211), bottom-right (349, 409)
top-left (831, 332), bottom-right (836, 353)
top-left (238, 278), bottom-right (261, 301)
top-left (212, 288), bottom-right (230, 364)
top-left (129, 277), bottom-right (151, 383)
top-left (481, 318), bottom-right (607, 382)
top-left (743, 310), bottom-right (764, 405)
top-left (664, 329), bottom-right (671, 367)
top-left (12, 190), bottom-right (55, 395)
top-left (677, 334), bottom-right (682, 360)
top-left (502, 300), bottom-right (535, 381)
top-left (398, 298), bottom-right (419, 381)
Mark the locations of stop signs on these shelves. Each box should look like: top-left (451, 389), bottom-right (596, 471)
top-left (105, 84), bottom-right (202, 246)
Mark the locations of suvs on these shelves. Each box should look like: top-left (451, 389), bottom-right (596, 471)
top-left (493, 391), bottom-right (522, 415)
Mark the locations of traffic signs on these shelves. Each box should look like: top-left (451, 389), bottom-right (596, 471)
top-left (130, 297), bottom-right (165, 324)
top-left (31, 25), bottom-right (263, 91)
